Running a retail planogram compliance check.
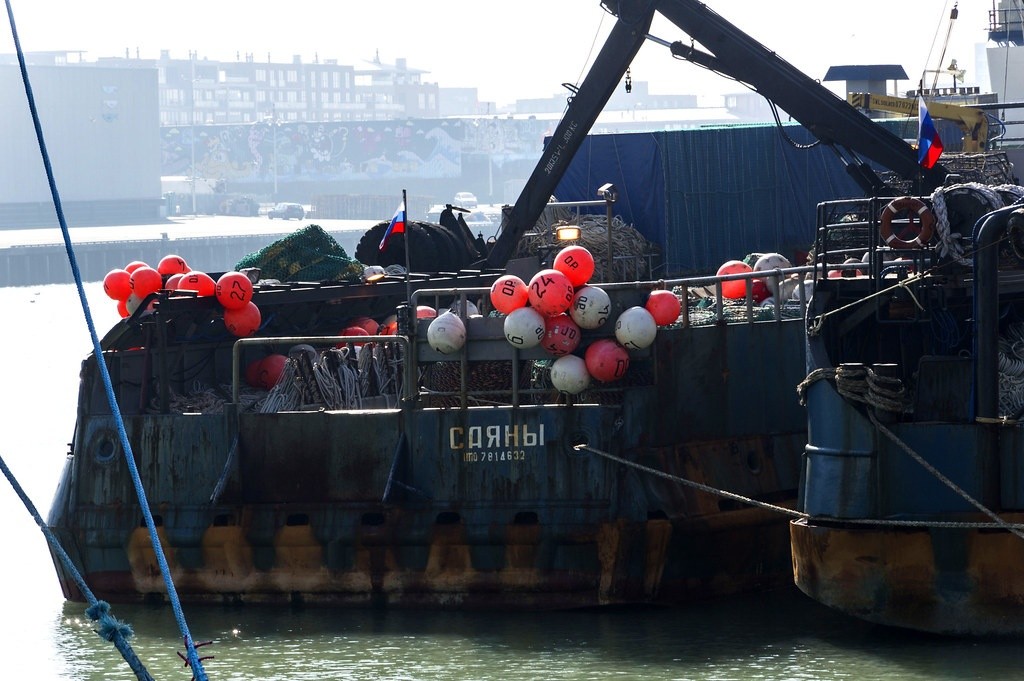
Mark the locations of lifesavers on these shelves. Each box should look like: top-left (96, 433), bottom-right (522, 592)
top-left (878, 196), bottom-right (936, 249)
top-left (86, 429), bottom-right (122, 467)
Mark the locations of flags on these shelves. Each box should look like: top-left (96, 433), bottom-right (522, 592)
top-left (917, 93), bottom-right (944, 170)
top-left (378, 202), bottom-right (405, 249)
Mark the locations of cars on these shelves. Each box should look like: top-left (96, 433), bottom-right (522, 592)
top-left (454, 192), bottom-right (477, 207)
top-left (268, 203), bottom-right (304, 220)
top-left (428, 208), bottom-right (442, 220)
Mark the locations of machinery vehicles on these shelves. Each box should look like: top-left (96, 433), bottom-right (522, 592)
top-left (482, 0), bottom-right (961, 270)
top-left (849, 91), bottom-right (1007, 155)
